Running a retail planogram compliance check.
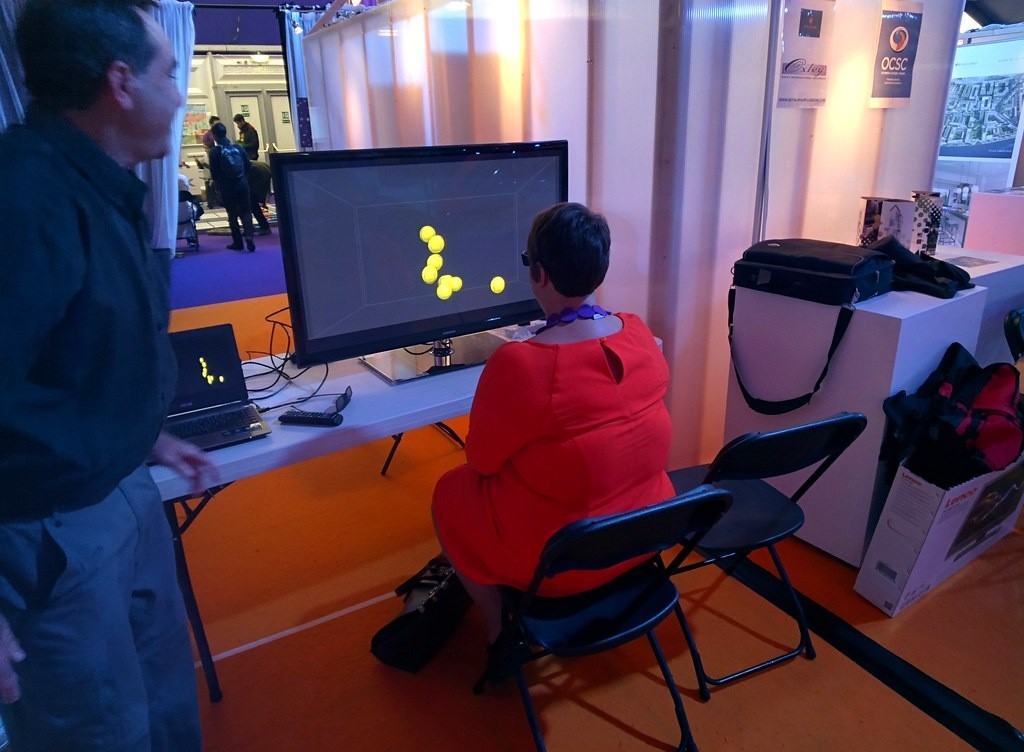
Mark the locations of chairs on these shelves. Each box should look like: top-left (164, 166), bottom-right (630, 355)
top-left (667, 410), bottom-right (868, 702)
top-left (473, 483), bottom-right (732, 752)
top-left (176, 190), bottom-right (200, 253)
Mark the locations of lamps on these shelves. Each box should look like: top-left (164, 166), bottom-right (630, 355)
top-left (956, 162), bottom-right (980, 201)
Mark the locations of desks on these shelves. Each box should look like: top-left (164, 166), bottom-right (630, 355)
top-left (931, 244), bottom-right (1024, 368)
top-left (148, 320), bottom-right (662, 700)
top-left (942, 206), bottom-right (968, 248)
top-left (723, 286), bottom-right (989, 569)
top-left (963, 190), bottom-right (1024, 275)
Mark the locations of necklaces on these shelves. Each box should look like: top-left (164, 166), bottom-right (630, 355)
top-left (535, 304), bottom-right (612, 334)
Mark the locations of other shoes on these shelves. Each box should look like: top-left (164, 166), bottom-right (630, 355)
top-left (472, 623), bottom-right (532, 695)
top-left (247, 242), bottom-right (255, 251)
top-left (226, 243), bottom-right (244, 250)
top-left (255, 229), bottom-right (271, 235)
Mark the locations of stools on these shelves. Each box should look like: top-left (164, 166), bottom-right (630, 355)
top-left (938, 215), bottom-right (958, 246)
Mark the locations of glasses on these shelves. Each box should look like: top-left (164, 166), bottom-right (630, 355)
top-left (521, 251), bottom-right (537, 267)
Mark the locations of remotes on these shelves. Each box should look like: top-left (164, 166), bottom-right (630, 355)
top-left (278, 410), bottom-right (344, 427)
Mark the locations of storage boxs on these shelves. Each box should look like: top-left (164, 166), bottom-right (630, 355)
top-left (853, 457), bottom-right (1024, 617)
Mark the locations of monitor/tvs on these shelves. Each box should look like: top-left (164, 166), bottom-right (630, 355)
top-left (269, 139), bottom-right (569, 382)
top-left (942, 459), bottom-right (1023, 560)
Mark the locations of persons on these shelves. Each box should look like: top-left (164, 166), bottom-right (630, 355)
top-left (0, 0), bottom-right (222, 752)
top-left (177, 173), bottom-right (200, 248)
top-left (432, 200), bottom-right (676, 695)
top-left (193, 114), bottom-right (272, 252)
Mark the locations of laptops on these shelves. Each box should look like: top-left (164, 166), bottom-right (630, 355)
top-left (159, 323), bottom-right (273, 452)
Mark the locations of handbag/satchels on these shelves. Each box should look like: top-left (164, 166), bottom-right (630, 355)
top-left (370, 549), bottom-right (473, 673)
top-left (887, 344), bottom-right (1022, 488)
top-left (735, 237), bottom-right (894, 305)
